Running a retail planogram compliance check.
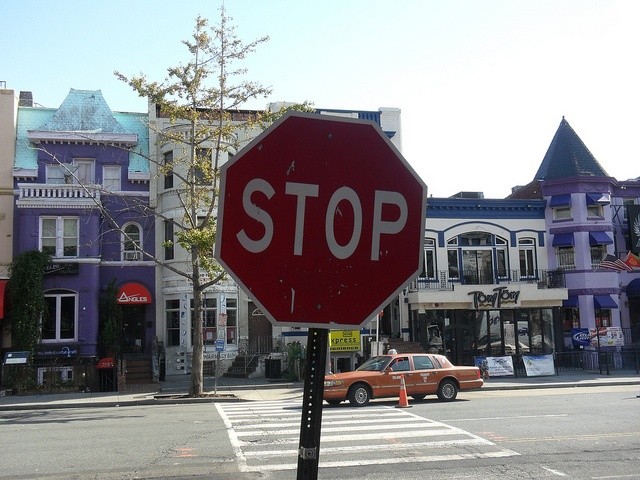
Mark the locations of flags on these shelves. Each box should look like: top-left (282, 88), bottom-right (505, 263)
top-left (627, 251), bottom-right (639, 270)
top-left (599, 253), bottom-right (632, 273)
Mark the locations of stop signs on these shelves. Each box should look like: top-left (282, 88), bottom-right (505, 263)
top-left (215, 111), bottom-right (428, 480)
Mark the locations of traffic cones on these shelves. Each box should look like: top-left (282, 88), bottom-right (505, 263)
top-left (399, 372), bottom-right (410, 407)
